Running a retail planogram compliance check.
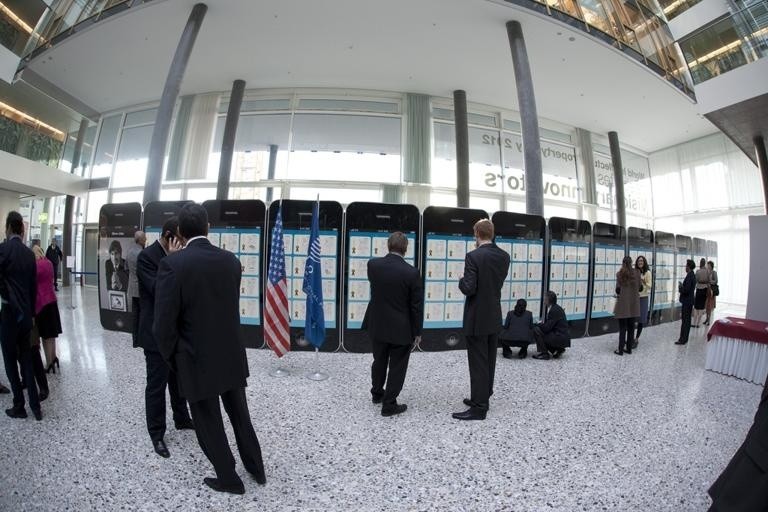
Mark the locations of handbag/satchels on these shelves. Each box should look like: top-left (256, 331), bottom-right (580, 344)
top-left (712, 284), bottom-right (719, 296)
top-left (706, 287), bottom-right (714, 312)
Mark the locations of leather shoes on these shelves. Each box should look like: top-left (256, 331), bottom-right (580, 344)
top-left (153, 440), bottom-right (170, 458)
top-left (1, 385), bottom-right (11, 393)
top-left (39, 391), bottom-right (49, 401)
top-left (452, 410), bottom-right (486, 420)
top-left (675, 339), bottom-right (687, 345)
top-left (175, 420), bottom-right (195, 430)
top-left (372, 390), bottom-right (407, 417)
top-left (463, 398), bottom-right (472, 407)
top-left (204, 477), bottom-right (246, 494)
top-left (532, 348), bottom-right (566, 360)
top-left (5, 408), bottom-right (27, 419)
top-left (614, 348), bottom-right (632, 355)
top-left (253, 473), bottom-right (266, 485)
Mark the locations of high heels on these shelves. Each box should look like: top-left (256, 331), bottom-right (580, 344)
top-left (46, 363), bottom-right (55, 374)
top-left (54, 357), bottom-right (60, 368)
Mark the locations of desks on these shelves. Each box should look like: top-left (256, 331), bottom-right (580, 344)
top-left (703, 315), bottom-right (768, 385)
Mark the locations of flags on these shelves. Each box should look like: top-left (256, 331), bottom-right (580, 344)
top-left (302, 200), bottom-right (326, 350)
top-left (263, 201), bottom-right (291, 358)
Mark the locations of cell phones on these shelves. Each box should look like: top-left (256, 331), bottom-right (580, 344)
top-left (491, 212), bottom-right (547, 347)
top-left (588, 221), bottom-right (718, 338)
top-left (98, 203), bottom-right (143, 333)
top-left (417, 207), bottom-right (489, 354)
top-left (266, 198), bottom-right (342, 352)
top-left (142, 200), bottom-right (194, 249)
top-left (544, 217), bottom-right (591, 339)
top-left (165, 233), bottom-right (177, 244)
top-left (200, 200), bottom-right (266, 348)
top-left (342, 201), bottom-right (422, 353)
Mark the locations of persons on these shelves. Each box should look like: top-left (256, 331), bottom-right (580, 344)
top-left (614, 256), bottom-right (644, 355)
top-left (361, 231), bottom-right (424, 416)
top-left (0, 211), bottom-right (63, 420)
top-left (151, 202), bottom-right (266, 494)
top-left (126, 231), bottom-right (146, 348)
top-left (135, 217), bottom-right (195, 458)
top-left (532, 290), bottom-right (571, 360)
top-left (106, 240), bottom-right (129, 291)
top-left (498, 299), bottom-right (534, 359)
top-left (625, 255), bottom-right (652, 349)
top-left (452, 218), bottom-right (510, 420)
top-left (675, 257), bottom-right (718, 345)
